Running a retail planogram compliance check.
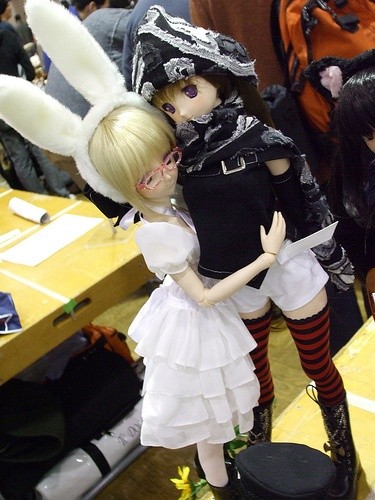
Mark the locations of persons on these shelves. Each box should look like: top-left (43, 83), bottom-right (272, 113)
top-left (0, 0), bottom-right (330, 196)
top-left (0, 0), bottom-right (375, 499)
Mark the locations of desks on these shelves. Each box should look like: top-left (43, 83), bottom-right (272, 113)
top-left (0, 187), bottom-right (153, 386)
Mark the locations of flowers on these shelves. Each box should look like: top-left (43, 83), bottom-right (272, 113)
top-left (169, 466), bottom-right (215, 500)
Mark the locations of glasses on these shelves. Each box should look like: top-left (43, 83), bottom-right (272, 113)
top-left (134, 147), bottom-right (183, 190)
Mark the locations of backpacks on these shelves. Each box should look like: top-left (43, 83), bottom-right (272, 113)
top-left (275, 0), bottom-right (375, 142)
